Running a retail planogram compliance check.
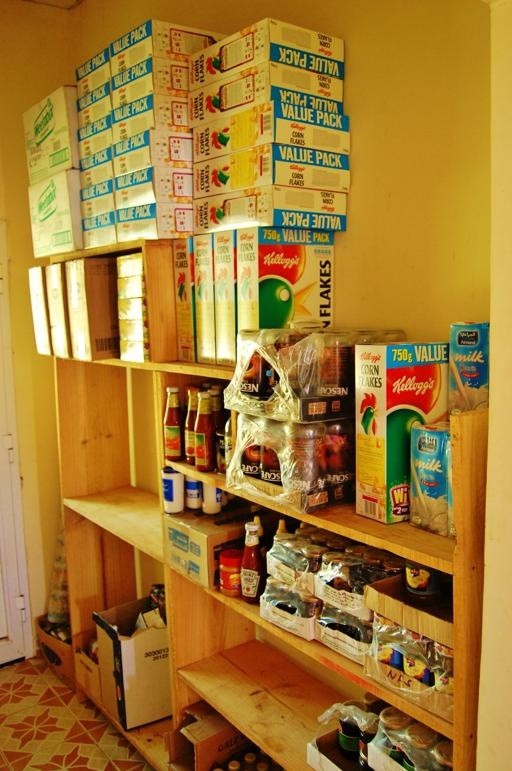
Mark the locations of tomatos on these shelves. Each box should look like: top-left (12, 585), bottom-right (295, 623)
top-left (188, 447), bottom-right (194, 454)
top-left (195, 446), bottom-right (205, 458)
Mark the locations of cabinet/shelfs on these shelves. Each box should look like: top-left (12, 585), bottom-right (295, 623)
top-left (53, 357), bottom-right (489, 771)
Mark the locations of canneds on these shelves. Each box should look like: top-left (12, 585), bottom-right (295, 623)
top-left (338, 691), bottom-right (453, 771)
top-left (240, 328), bottom-right (406, 487)
top-left (219, 549), bottom-right (244, 596)
top-left (264, 527), bottom-right (405, 643)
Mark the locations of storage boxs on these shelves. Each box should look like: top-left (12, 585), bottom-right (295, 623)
top-left (34, 595), bottom-right (173, 731)
top-left (162, 501), bottom-right (296, 591)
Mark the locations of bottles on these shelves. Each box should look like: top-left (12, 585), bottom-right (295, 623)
top-left (336, 700), bottom-right (453, 771)
top-left (212, 744), bottom-right (284, 770)
top-left (161, 378), bottom-right (231, 473)
top-left (148, 466), bottom-right (453, 701)
top-left (239, 329), bottom-right (415, 492)
